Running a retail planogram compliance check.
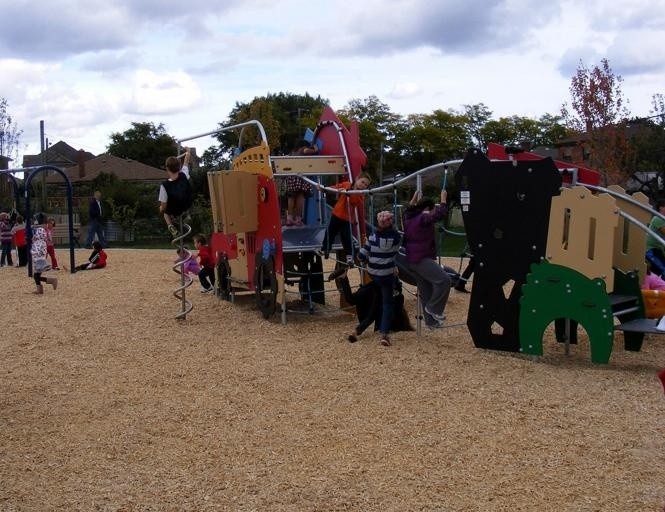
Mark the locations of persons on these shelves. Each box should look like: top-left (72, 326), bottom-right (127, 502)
top-left (193, 233), bottom-right (215, 293)
top-left (158, 146), bottom-right (197, 236)
top-left (174, 245), bottom-right (200, 275)
top-left (0, 211), bottom-right (58, 294)
top-left (645, 198), bottom-right (665, 281)
top-left (86, 189), bottom-right (106, 248)
top-left (286, 139), bottom-right (318, 226)
top-left (63, 241), bottom-right (107, 273)
top-left (317, 172), bottom-right (474, 345)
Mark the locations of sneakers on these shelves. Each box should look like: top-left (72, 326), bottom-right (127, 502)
top-left (201, 285), bottom-right (214, 293)
top-left (285, 215), bottom-right (470, 346)
top-left (168, 224), bottom-right (180, 238)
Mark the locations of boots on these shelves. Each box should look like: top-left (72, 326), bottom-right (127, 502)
top-left (46, 278), bottom-right (57, 290)
top-left (32, 285), bottom-right (44, 294)
